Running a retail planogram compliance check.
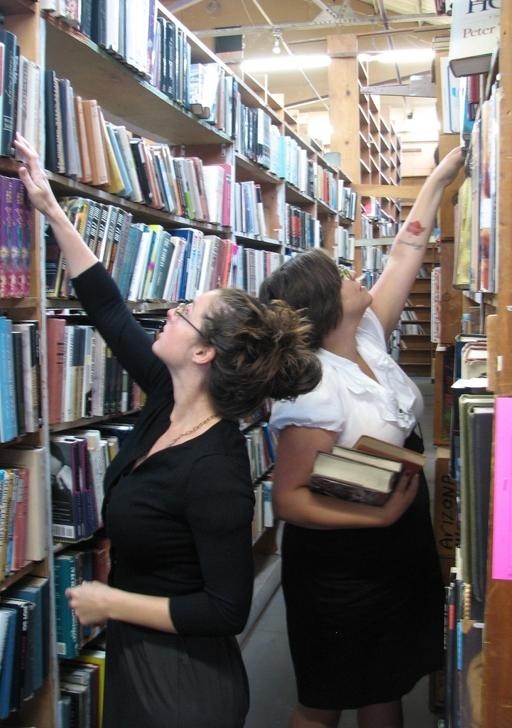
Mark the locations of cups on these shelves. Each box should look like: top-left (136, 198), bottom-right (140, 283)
top-left (175, 302), bottom-right (213, 345)
top-left (339, 264), bottom-right (352, 281)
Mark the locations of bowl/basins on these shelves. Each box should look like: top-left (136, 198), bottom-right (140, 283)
top-left (142, 414), bottom-right (219, 458)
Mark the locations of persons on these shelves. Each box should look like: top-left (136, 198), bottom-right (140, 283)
top-left (13, 132), bottom-right (324, 728)
top-left (259, 144), bottom-right (446, 728)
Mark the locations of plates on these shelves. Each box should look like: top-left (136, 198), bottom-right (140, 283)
top-left (440, 5), bottom-right (509, 720)
top-left (0, 3), bottom-right (61, 722)
top-left (396, 175), bottom-right (438, 376)
top-left (39, 0), bottom-right (237, 723)
top-left (328, 34), bottom-right (401, 288)
top-left (336, 171), bottom-right (354, 285)
top-left (238, 71), bottom-right (340, 563)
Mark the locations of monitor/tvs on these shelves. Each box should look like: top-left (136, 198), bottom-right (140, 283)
top-left (48, 72), bottom-right (233, 229)
top-left (1, 314), bottom-right (41, 444)
top-left (405, 298), bottom-right (413, 307)
top-left (58, 642), bottom-right (107, 728)
top-left (462, 72), bottom-right (500, 147)
top-left (444, 585), bottom-right (485, 728)
top-left (241, 421), bottom-right (279, 481)
top-left (1, 174), bottom-right (32, 300)
top-left (48, 429), bottom-right (121, 544)
top-left (334, 225), bottom-right (355, 261)
top-left (338, 180), bottom-right (357, 221)
top-left (107, 346), bottom-right (145, 423)
top-left (452, 147), bottom-right (498, 297)
top-left (124, 213), bottom-right (286, 303)
top-left (283, 135), bottom-right (338, 210)
top-left (45, 190), bottom-right (131, 302)
top-left (309, 450), bottom-right (395, 507)
top-left (399, 311), bottom-right (418, 320)
top-left (394, 324), bottom-right (423, 335)
top-left (440, 57), bottom-right (464, 135)
top-left (447, 0), bottom-right (502, 77)
top-left (359, 246), bottom-right (389, 272)
top-left (44, 317), bottom-right (105, 426)
top-left (0, 575), bottom-right (49, 726)
top-left (416, 266), bottom-right (427, 278)
top-left (432, 32), bottom-right (449, 52)
top-left (52, 540), bottom-right (112, 659)
top-left (37, 0), bottom-right (241, 141)
top-left (234, 179), bottom-right (267, 238)
top-left (452, 332), bottom-right (512, 602)
top-left (285, 203), bottom-right (321, 251)
top-left (361, 218), bottom-right (405, 238)
top-left (434, 0), bottom-right (451, 15)
top-left (240, 103), bottom-right (285, 180)
top-left (0, 30), bottom-right (46, 165)
top-left (0, 444), bottom-right (48, 579)
top-left (360, 195), bottom-right (381, 219)
top-left (252, 468), bottom-right (274, 543)
top-left (331, 446), bottom-right (403, 474)
top-left (351, 436), bottom-right (425, 479)
top-left (134, 313), bottom-right (164, 341)
top-left (362, 273), bottom-right (382, 290)
